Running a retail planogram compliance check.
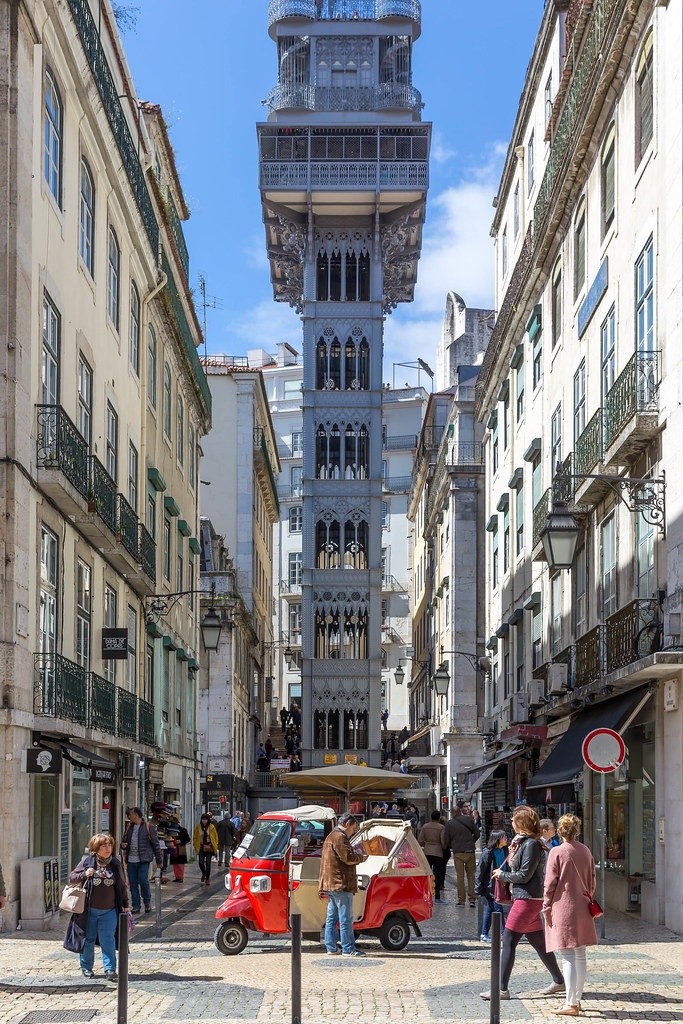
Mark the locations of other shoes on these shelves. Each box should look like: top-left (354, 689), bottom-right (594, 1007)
top-left (201, 874), bottom-right (205, 882)
top-left (435, 899), bottom-right (449, 904)
top-left (470, 902), bottom-right (475, 906)
top-left (550, 1001), bottom-right (581, 1016)
top-left (540, 980), bottom-right (566, 993)
top-left (480, 989), bottom-right (511, 1000)
top-left (481, 934), bottom-right (492, 943)
top-left (455, 903), bottom-right (465, 907)
top-left (206, 880), bottom-right (210, 885)
top-left (172, 879), bottom-right (183, 883)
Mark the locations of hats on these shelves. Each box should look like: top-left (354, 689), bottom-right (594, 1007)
top-left (151, 801), bottom-right (183, 849)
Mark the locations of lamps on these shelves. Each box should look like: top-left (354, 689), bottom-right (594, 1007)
top-left (394, 658), bottom-right (432, 684)
top-left (538, 463), bottom-right (667, 570)
top-left (260, 637), bottom-right (294, 663)
top-left (144, 582), bottom-right (223, 654)
top-left (433, 645), bottom-right (492, 698)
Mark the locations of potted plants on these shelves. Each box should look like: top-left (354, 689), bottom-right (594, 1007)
top-left (116, 523), bottom-right (133, 543)
top-left (44, 452), bottom-right (58, 470)
top-left (88, 500), bottom-right (102, 512)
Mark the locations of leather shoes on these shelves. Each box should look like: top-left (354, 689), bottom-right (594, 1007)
top-left (131, 907), bottom-right (140, 914)
top-left (82, 968), bottom-right (94, 977)
top-left (106, 970), bottom-right (118, 978)
top-left (145, 903), bottom-right (151, 913)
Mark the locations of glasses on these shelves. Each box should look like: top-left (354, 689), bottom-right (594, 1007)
top-left (510, 816), bottom-right (513, 822)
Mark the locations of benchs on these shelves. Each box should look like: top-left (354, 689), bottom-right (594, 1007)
top-left (355, 855), bottom-right (398, 886)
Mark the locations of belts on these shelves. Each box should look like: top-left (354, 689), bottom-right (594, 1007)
top-left (462, 852), bottom-right (475, 853)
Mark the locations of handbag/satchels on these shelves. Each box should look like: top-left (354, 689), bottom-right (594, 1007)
top-left (589, 899), bottom-right (604, 918)
top-left (125, 911), bottom-right (135, 932)
top-left (202, 843), bottom-right (213, 853)
top-left (494, 876), bottom-right (513, 906)
top-left (59, 877), bottom-right (89, 914)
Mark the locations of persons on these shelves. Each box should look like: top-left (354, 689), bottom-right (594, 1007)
top-left (62, 833), bottom-right (131, 981)
top-left (256, 700), bottom-right (302, 772)
top-left (381, 708), bottom-right (411, 774)
top-left (457, 800), bottom-right (473, 817)
top-left (356, 758), bottom-right (368, 768)
top-left (318, 812), bottom-right (369, 957)
top-left (163, 804), bottom-right (263, 885)
top-left (193, 813), bottom-right (219, 885)
top-left (120, 807), bottom-right (164, 913)
top-left (418, 806), bottom-right (596, 1016)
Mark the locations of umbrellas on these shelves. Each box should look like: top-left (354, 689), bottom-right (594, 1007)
top-left (280, 761), bottom-right (424, 815)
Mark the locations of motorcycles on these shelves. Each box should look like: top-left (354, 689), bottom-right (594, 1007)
top-left (213, 804), bottom-right (435, 956)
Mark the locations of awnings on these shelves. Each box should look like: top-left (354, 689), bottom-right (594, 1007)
top-left (39, 734), bottom-right (116, 784)
top-left (398, 727), bottom-right (431, 752)
top-left (456, 749), bottom-right (527, 775)
top-left (467, 744), bottom-right (517, 793)
top-left (526, 682), bottom-right (653, 789)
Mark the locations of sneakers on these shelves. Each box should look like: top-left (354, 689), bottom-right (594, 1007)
top-left (342, 948), bottom-right (366, 956)
top-left (327, 945), bottom-right (342, 955)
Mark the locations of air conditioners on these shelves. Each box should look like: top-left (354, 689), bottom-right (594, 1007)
top-left (122, 752), bottom-right (141, 780)
top-left (526, 679), bottom-right (544, 706)
top-left (509, 693), bottom-right (530, 725)
top-left (547, 663), bottom-right (568, 696)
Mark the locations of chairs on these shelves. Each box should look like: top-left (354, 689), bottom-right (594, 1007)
top-left (299, 857), bottom-right (321, 880)
